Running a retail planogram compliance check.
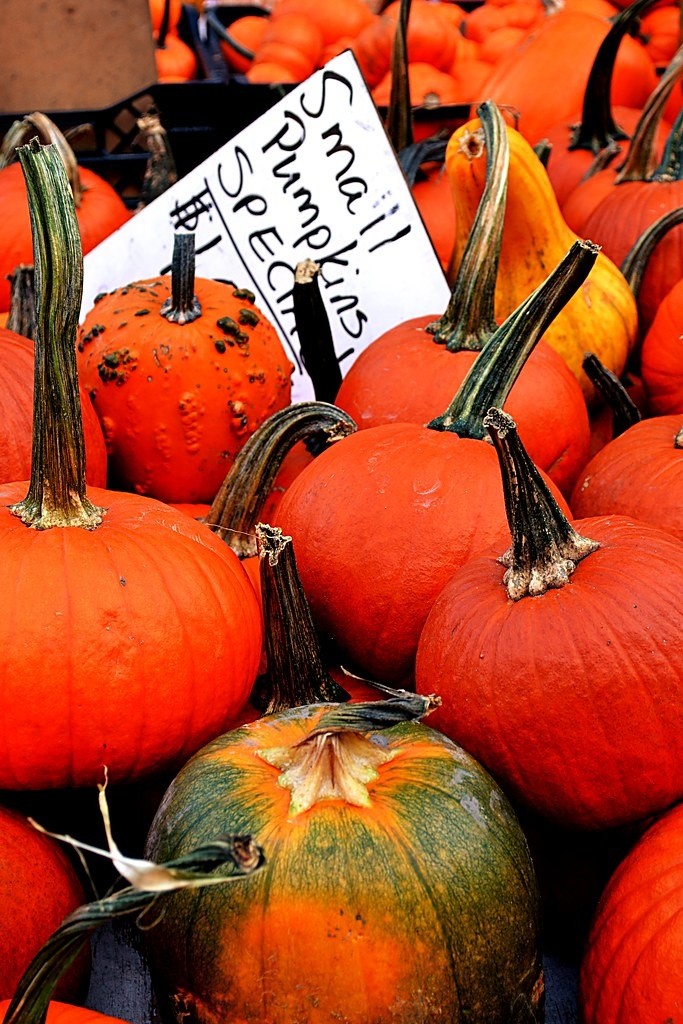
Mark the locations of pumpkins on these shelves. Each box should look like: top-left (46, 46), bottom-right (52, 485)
top-left (0, 0), bottom-right (683, 1024)
